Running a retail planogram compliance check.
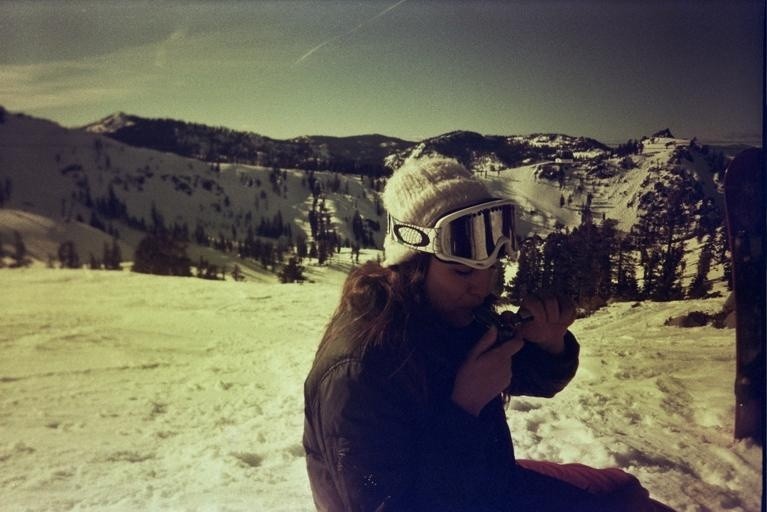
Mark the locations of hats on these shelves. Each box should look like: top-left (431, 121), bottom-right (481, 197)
top-left (381, 146), bottom-right (490, 271)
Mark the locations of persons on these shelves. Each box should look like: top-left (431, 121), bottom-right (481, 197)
top-left (303, 158), bottom-right (677, 512)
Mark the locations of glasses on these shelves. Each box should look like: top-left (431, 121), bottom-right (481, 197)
top-left (389, 198), bottom-right (518, 269)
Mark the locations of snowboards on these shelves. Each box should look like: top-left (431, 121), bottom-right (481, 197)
top-left (724, 146), bottom-right (766, 445)
